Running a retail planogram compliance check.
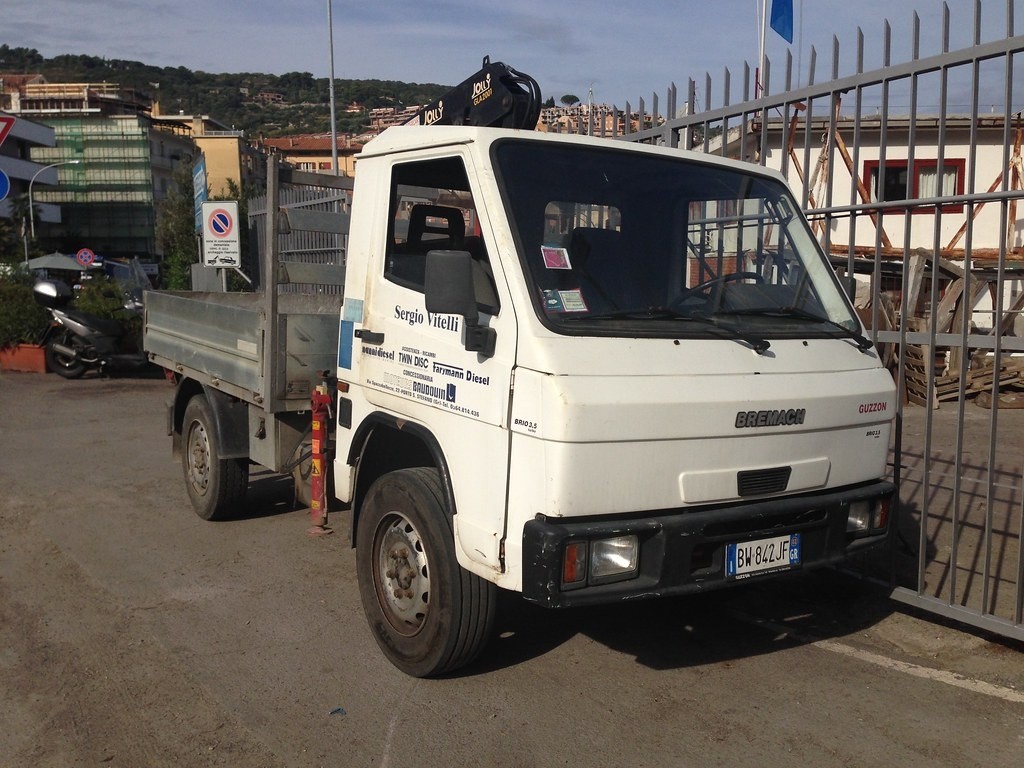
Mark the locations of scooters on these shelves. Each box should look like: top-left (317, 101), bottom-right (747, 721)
top-left (33, 280), bottom-right (180, 383)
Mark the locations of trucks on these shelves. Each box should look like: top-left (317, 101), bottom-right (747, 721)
top-left (141, 120), bottom-right (902, 680)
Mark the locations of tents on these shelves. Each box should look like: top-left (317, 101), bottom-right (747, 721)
top-left (22, 252), bottom-right (94, 271)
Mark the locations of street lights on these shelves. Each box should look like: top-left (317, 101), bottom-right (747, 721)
top-left (29, 159), bottom-right (79, 237)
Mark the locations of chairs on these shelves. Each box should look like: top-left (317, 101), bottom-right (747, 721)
top-left (389, 227), bottom-right (635, 312)
